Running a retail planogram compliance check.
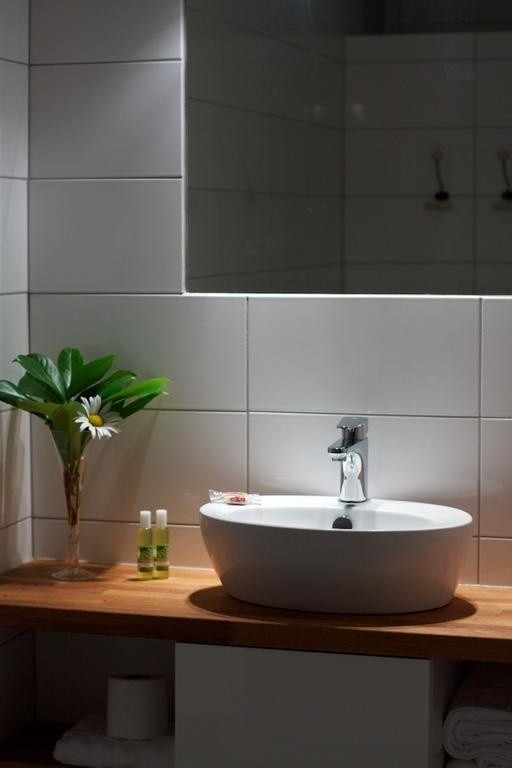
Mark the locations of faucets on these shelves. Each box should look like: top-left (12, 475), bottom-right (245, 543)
top-left (328, 417), bottom-right (369, 502)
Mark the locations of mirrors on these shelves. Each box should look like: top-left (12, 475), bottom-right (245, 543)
top-left (178, 0), bottom-right (512, 301)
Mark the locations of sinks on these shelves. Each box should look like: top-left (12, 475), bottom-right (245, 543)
top-left (198, 495), bottom-right (472, 615)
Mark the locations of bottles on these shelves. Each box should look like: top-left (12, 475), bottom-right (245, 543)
top-left (136, 508), bottom-right (169, 581)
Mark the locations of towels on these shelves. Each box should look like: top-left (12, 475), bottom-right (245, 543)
top-left (443, 706), bottom-right (512, 768)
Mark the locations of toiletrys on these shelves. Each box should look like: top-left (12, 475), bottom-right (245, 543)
top-left (136, 508), bottom-right (170, 581)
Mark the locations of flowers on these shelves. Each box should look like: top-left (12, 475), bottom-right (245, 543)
top-left (0, 348), bottom-right (170, 545)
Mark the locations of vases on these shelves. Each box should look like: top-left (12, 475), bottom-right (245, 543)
top-left (49, 431), bottom-right (98, 583)
